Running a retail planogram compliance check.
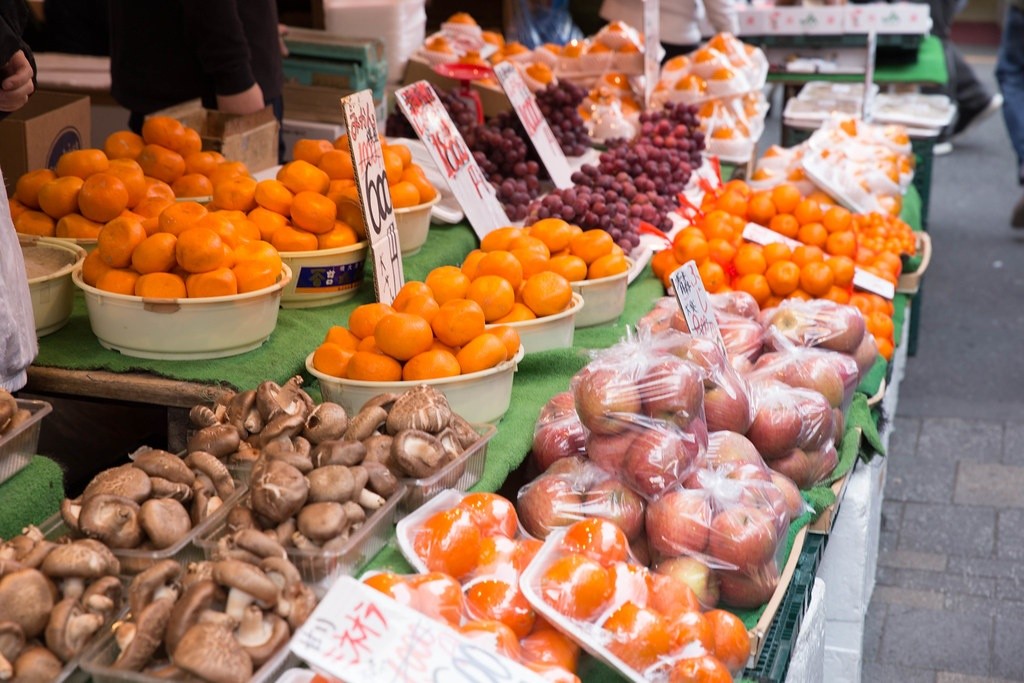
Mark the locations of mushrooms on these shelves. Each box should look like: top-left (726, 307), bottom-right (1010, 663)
top-left (0, 376), bottom-right (484, 682)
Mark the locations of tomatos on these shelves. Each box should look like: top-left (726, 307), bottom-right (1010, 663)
top-left (303, 490), bottom-right (752, 683)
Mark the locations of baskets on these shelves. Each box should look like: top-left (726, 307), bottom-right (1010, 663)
top-left (741, 534), bottom-right (825, 683)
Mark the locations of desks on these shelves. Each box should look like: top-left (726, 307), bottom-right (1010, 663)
top-left (770, 35), bottom-right (947, 99)
top-left (785, 97), bottom-right (960, 358)
top-left (27, 221), bottom-right (477, 460)
top-left (1, 455), bottom-right (66, 546)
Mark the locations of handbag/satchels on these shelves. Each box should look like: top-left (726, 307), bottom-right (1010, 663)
top-left (516, 290), bottom-right (880, 617)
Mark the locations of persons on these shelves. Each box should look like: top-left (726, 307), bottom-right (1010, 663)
top-left (592, 0), bottom-right (740, 65)
top-left (996, 0), bottom-right (1024, 230)
top-left (107, 0), bottom-right (326, 142)
top-left (0, 0), bottom-right (38, 204)
top-left (908, 0), bottom-right (995, 157)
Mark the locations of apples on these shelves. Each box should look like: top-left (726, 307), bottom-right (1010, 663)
top-left (516, 290), bottom-right (877, 615)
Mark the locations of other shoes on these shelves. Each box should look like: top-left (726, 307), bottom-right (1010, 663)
top-left (932, 142), bottom-right (952, 155)
top-left (939, 94), bottom-right (1003, 146)
top-left (1008, 195), bottom-right (1024, 227)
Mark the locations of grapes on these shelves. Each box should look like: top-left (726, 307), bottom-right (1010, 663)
top-left (387, 78), bottom-right (707, 255)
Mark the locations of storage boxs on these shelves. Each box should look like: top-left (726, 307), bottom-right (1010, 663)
top-left (285, 96), bottom-right (388, 164)
top-left (0, 391), bottom-right (495, 683)
top-left (144, 98), bottom-right (281, 175)
top-left (0, 92), bottom-right (92, 197)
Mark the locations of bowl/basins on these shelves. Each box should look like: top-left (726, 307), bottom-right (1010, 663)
top-left (278, 241), bottom-right (370, 309)
top-left (17, 235), bottom-right (82, 338)
top-left (305, 350), bottom-right (525, 428)
top-left (72, 262), bottom-right (293, 361)
top-left (393, 188), bottom-right (442, 258)
top-left (571, 256), bottom-right (636, 330)
top-left (18, 231), bottom-right (98, 291)
top-left (485, 292), bottom-right (584, 353)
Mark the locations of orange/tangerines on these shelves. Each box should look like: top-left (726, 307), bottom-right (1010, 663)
top-left (7, 114), bottom-right (628, 384)
top-left (648, 181), bottom-right (913, 362)
top-left (749, 117), bottom-right (913, 217)
top-left (420, 14), bottom-right (770, 143)
